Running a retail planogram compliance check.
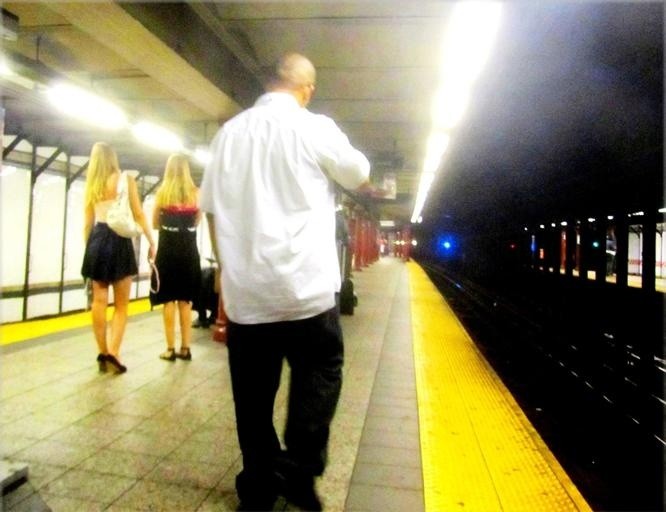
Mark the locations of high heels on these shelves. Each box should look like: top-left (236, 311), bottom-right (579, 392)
top-left (105, 353), bottom-right (128, 376)
top-left (97, 352), bottom-right (107, 372)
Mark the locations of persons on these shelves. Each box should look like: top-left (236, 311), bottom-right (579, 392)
top-left (151, 154), bottom-right (202, 361)
top-left (191, 214), bottom-right (218, 327)
top-left (81, 141), bottom-right (154, 373)
top-left (200, 53), bottom-right (374, 512)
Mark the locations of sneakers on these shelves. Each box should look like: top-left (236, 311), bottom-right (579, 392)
top-left (190, 317), bottom-right (212, 328)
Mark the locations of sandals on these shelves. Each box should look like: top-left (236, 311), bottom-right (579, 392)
top-left (175, 347), bottom-right (192, 360)
top-left (159, 348), bottom-right (177, 361)
